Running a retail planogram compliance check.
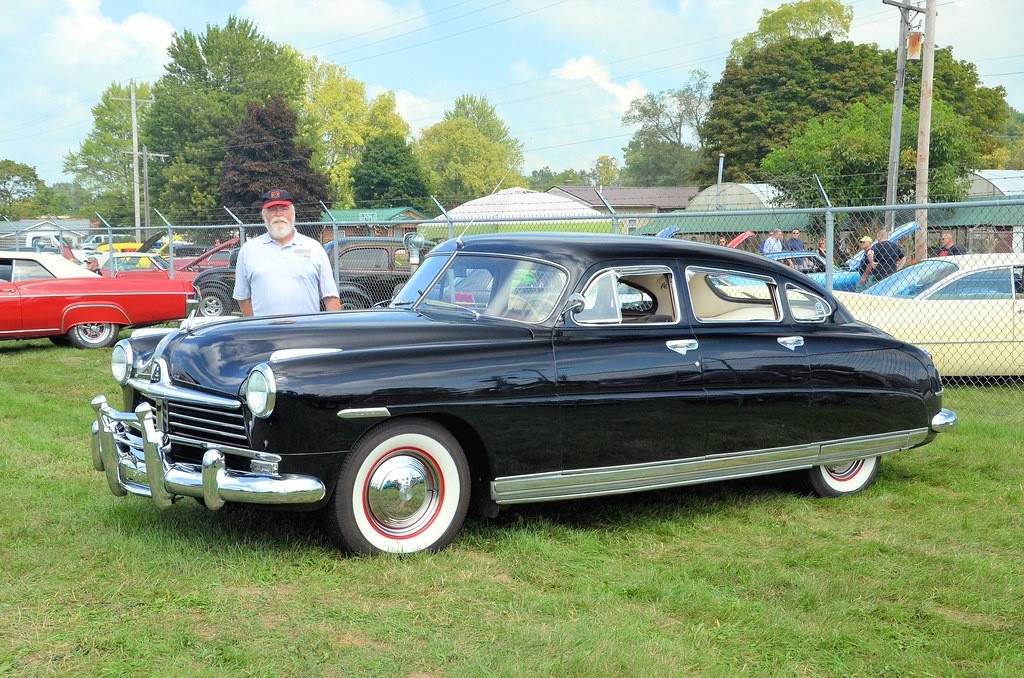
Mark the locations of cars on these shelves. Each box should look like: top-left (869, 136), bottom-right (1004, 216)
top-left (88, 232), bottom-right (958, 562)
top-left (66, 231), bottom-right (241, 285)
top-left (1, 236), bottom-right (201, 350)
top-left (709, 222), bottom-right (920, 295)
top-left (718, 251), bottom-right (1024, 379)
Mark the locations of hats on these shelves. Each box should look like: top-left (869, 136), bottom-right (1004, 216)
top-left (84, 257), bottom-right (97, 262)
top-left (859, 235), bottom-right (873, 242)
top-left (261, 190), bottom-right (294, 209)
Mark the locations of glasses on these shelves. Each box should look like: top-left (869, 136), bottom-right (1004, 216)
top-left (793, 232), bottom-right (799, 234)
top-left (86, 262), bottom-right (91, 265)
top-left (939, 238), bottom-right (947, 241)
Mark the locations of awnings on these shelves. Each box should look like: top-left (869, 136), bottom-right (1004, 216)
top-left (629, 194), bottom-right (1023, 236)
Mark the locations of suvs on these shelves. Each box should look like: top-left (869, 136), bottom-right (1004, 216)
top-left (194, 235), bottom-right (440, 322)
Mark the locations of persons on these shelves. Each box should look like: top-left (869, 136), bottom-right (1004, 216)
top-left (214, 239), bottom-right (220, 246)
top-left (718, 230), bottom-right (960, 294)
top-left (84, 257), bottom-right (102, 276)
top-left (232, 188), bottom-right (341, 317)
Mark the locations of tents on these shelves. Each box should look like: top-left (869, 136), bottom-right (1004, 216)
top-left (416, 185), bottom-right (623, 244)
